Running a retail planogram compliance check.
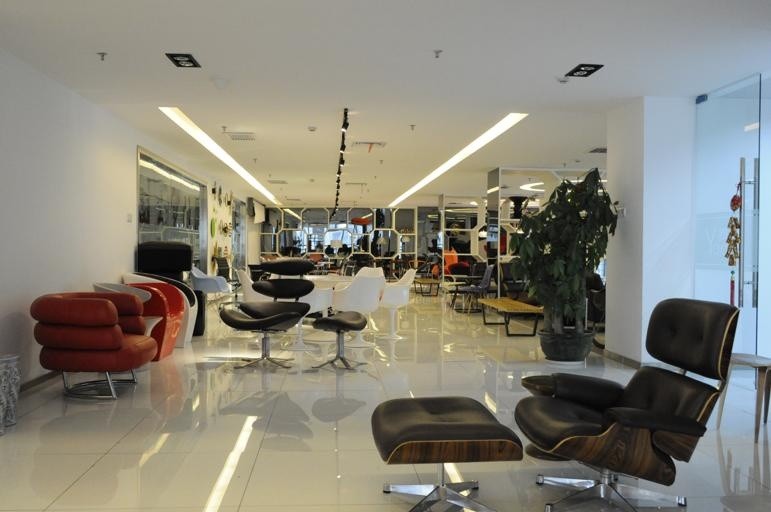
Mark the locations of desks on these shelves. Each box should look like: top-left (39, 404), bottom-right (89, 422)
top-left (715, 352), bottom-right (771, 443)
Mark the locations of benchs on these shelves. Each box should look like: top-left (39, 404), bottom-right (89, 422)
top-left (478, 297), bottom-right (544, 337)
top-left (414, 279), bottom-right (441, 297)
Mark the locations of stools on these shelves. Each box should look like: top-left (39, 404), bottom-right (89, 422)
top-left (372, 396), bottom-right (522, 512)
top-left (310, 311), bottom-right (367, 372)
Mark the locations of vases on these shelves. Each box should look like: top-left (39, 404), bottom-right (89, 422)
top-left (0, 392), bottom-right (7, 436)
top-left (0, 354), bottom-right (22, 436)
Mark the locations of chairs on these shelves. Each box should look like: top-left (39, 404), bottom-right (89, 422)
top-left (29, 292), bottom-right (157, 401)
top-left (449, 264), bottom-right (496, 313)
top-left (325, 266), bottom-right (384, 347)
top-left (514, 298), bottom-right (741, 512)
top-left (236, 270), bottom-right (335, 352)
top-left (221, 257), bottom-right (315, 369)
top-left (123, 272), bottom-right (198, 346)
top-left (122, 281), bottom-right (184, 362)
top-left (378, 268), bottom-right (417, 340)
top-left (137, 241), bottom-right (208, 336)
top-left (92, 282), bottom-right (162, 372)
top-left (187, 262), bottom-right (232, 328)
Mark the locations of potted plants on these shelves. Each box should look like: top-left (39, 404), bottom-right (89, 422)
top-left (511, 168), bottom-right (617, 360)
